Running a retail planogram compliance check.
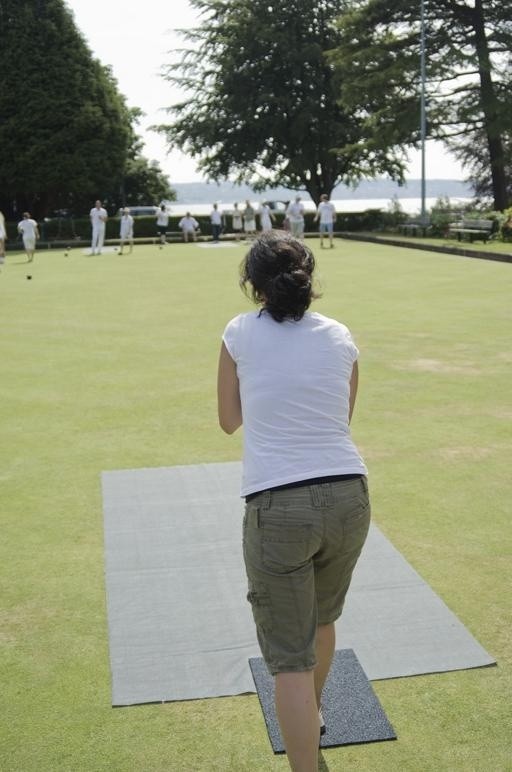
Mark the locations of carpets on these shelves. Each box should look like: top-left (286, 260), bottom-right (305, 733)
top-left (102, 459), bottom-right (494, 708)
top-left (246, 647), bottom-right (399, 752)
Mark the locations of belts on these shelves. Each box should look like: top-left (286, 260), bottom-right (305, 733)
top-left (245, 474), bottom-right (362, 503)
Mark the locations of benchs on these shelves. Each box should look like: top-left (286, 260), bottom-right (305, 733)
top-left (397, 217), bottom-right (428, 237)
top-left (159, 226), bottom-right (201, 242)
top-left (448, 217), bottom-right (495, 243)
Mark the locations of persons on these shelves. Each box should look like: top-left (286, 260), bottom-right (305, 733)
top-left (178, 211), bottom-right (200, 243)
top-left (154, 204), bottom-right (170, 244)
top-left (0, 210), bottom-right (9, 265)
top-left (217, 231), bottom-right (372, 772)
top-left (207, 194), bottom-right (339, 250)
top-left (116, 206), bottom-right (135, 255)
top-left (17, 211), bottom-right (41, 263)
top-left (87, 200), bottom-right (109, 257)
